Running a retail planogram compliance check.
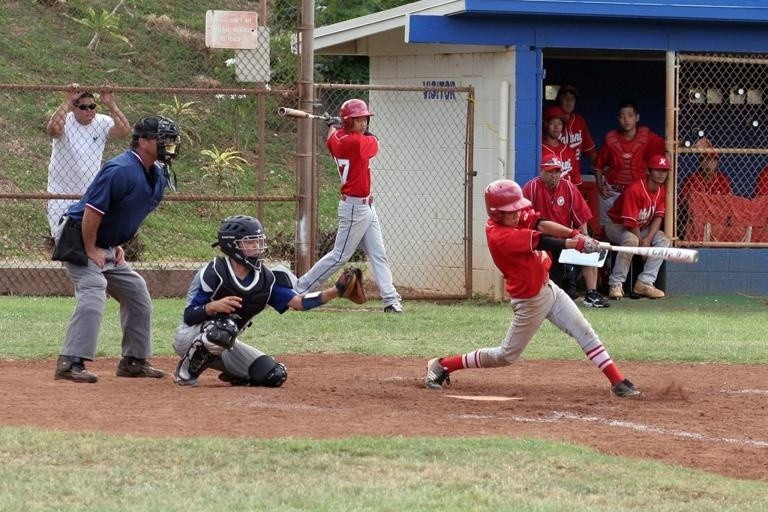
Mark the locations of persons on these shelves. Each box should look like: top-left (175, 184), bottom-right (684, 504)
top-left (273, 97), bottom-right (404, 314)
top-left (681, 152), bottom-right (733, 209)
top-left (46, 84), bottom-right (133, 250)
top-left (170, 216), bottom-right (366, 391)
top-left (523, 85), bottom-right (673, 309)
top-left (424, 179), bottom-right (647, 401)
top-left (50, 115), bottom-right (183, 383)
top-left (756, 164), bottom-right (768, 198)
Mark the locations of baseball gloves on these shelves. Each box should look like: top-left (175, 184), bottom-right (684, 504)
top-left (336, 265), bottom-right (368, 304)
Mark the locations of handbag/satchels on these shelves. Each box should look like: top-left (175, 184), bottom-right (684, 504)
top-left (686, 192), bottom-right (767, 241)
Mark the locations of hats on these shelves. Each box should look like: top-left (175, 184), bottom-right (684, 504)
top-left (559, 82), bottom-right (578, 98)
top-left (543, 108), bottom-right (564, 123)
top-left (701, 147), bottom-right (719, 160)
top-left (539, 154), bottom-right (562, 172)
top-left (648, 156), bottom-right (670, 170)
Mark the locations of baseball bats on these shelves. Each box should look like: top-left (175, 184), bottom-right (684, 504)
top-left (277, 107), bottom-right (340, 121)
top-left (598, 244), bottom-right (699, 264)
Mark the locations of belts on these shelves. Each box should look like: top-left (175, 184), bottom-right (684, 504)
top-left (604, 182), bottom-right (625, 193)
top-left (341, 196), bottom-right (374, 204)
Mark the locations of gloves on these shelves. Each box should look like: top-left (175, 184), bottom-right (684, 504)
top-left (575, 238), bottom-right (599, 254)
top-left (568, 230), bottom-right (587, 239)
top-left (322, 112), bottom-right (333, 126)
top-left (332, 116), bottom-right (341, 129)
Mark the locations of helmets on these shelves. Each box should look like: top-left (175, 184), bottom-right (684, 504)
top-left (341, 99), bottom-right (375, 130)
top-left (219, 215), bottom-right (268, 266)
top-left (133, 115), bottom-right (182, 144)
top-left (485, 180), bottom-right (532, 224)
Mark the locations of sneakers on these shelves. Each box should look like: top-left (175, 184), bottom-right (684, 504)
top-left (383, 304), bottom-right (402, 313)
top-left (611, 380), bottom-right (644, 400)
top-left (584, 290), bottom-right (610, 308)
top-left (426, 358), bottom-right (450, 390)
top-left (55, 357), bottom-right (99, 382)
top-left (117, 358), bottom-right (164, 377)
top-left (633, 280), bottom-right (665, 298)
top-left (609, 285), bottom-right (625, 300)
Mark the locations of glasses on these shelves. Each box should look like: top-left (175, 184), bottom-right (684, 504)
top-left (75, 103), bottom-right (98, 110)
